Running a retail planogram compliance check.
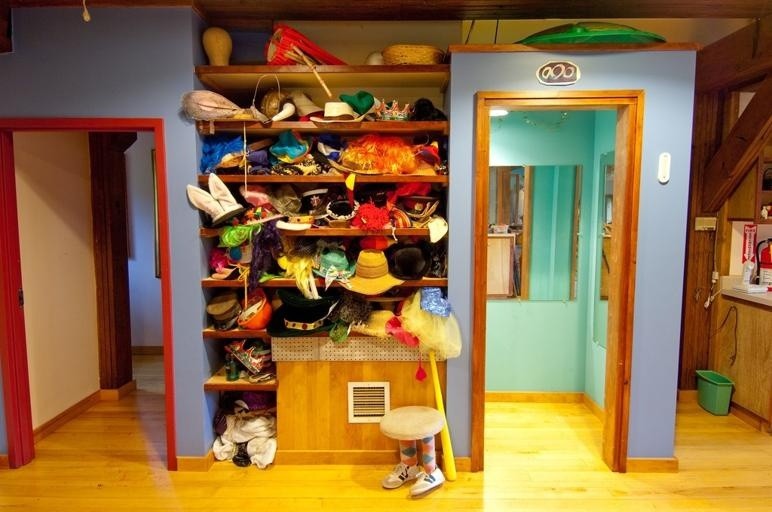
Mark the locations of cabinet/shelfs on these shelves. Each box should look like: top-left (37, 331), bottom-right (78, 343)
top-left (195, 19), bottom-right (450, 473)
top-left (488, 232), bottom-right (522, 299)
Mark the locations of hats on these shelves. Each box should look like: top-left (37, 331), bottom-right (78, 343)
top-left (234, 390), bottom-right (276, 411)
top-left (186, 90), bottom-right (447, 337)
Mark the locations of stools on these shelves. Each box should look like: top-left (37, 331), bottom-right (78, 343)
top-left (379, 407), bottom-right (447, 498)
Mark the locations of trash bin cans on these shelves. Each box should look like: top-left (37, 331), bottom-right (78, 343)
top-left (695, 370), bottom-right (734, 416)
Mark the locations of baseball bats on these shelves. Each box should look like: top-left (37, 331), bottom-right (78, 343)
top-left (430, 351), bottom-right (456, 479)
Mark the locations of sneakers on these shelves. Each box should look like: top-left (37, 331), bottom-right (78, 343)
top-left (382, 462), bottom-right (420, 489)
top-left (410, 465), bottom-right (445, 497)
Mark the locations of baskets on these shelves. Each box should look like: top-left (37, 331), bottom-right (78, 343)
top-left (381, 44), bottom-right (445, 65)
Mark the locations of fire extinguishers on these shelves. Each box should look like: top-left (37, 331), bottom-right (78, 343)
top-left (756, 238), bottom-right (772, 292)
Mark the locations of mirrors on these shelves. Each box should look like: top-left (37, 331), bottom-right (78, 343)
top-left (594, 152), bottom-right (615, 349)
top-left (488, 166), bottom-right (580, 301)
